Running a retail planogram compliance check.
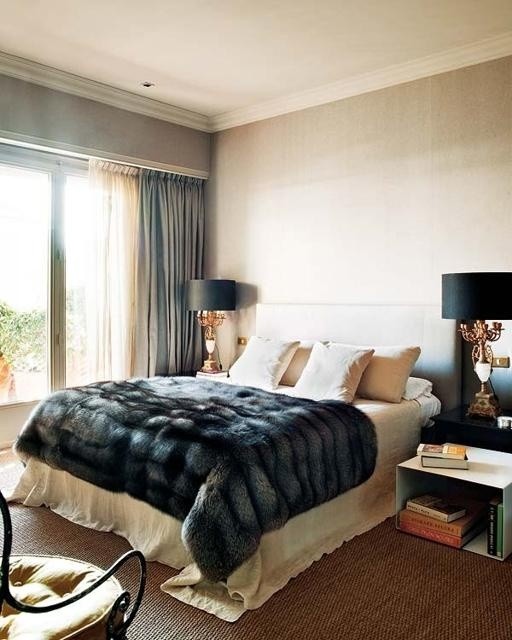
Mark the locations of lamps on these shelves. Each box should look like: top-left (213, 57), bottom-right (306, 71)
top-left (440, 270), bottom-right (512, 420)
top-left (184, 277), bottom-right (240, 375)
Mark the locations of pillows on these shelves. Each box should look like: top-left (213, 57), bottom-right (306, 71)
top-left (357, 344), bottom-right (424, 405)
top-left (278, 343), bottom-right (315, 388)
top-left (226, 335), bottom-right (301, 391)
top-left (290, 340), bottom-right (375, 407)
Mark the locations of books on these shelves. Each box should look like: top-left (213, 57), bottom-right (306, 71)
top-left (399, 487), bottom-right (504, 559)
top-left (197, 371), bottom-right (227, 377)
top-left (417, 443), bottom-right (467, 460)
top-left (421, 454), bottom-right (469, 470)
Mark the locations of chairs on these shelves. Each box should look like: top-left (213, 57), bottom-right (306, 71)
top-left (0, 489), bottom-right (147, 640)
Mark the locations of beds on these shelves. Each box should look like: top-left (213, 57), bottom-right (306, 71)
top-left (7, 300), bottom-right (466, 626)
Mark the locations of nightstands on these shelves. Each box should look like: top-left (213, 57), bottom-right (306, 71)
top-left (393, 442), bottom-right (512, 561)
top-left (427, 400), bottom-right (512, 455)
top-left (194, 371), bottom-right (227, 380)
